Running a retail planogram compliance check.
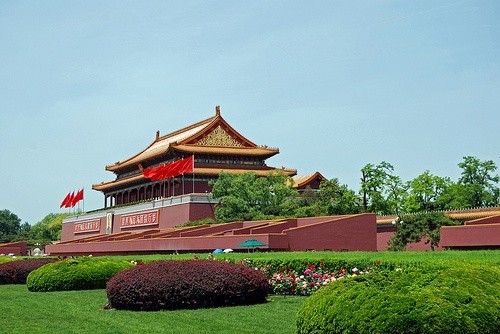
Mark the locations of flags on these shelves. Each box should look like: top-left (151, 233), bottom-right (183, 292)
top-left (72, 190), bottom-right (79, 207)
top-left (65, 192), bottom-right (74, 208)
top-left (72, 189), bottom-right (83, 203)
top-left (143, 155), bottom-right (193, 181)
top-left (60, 193), bottom-right (70, 209)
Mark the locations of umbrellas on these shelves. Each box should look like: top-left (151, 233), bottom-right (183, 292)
top-left (232, 239), bottom-right (268, 253)
